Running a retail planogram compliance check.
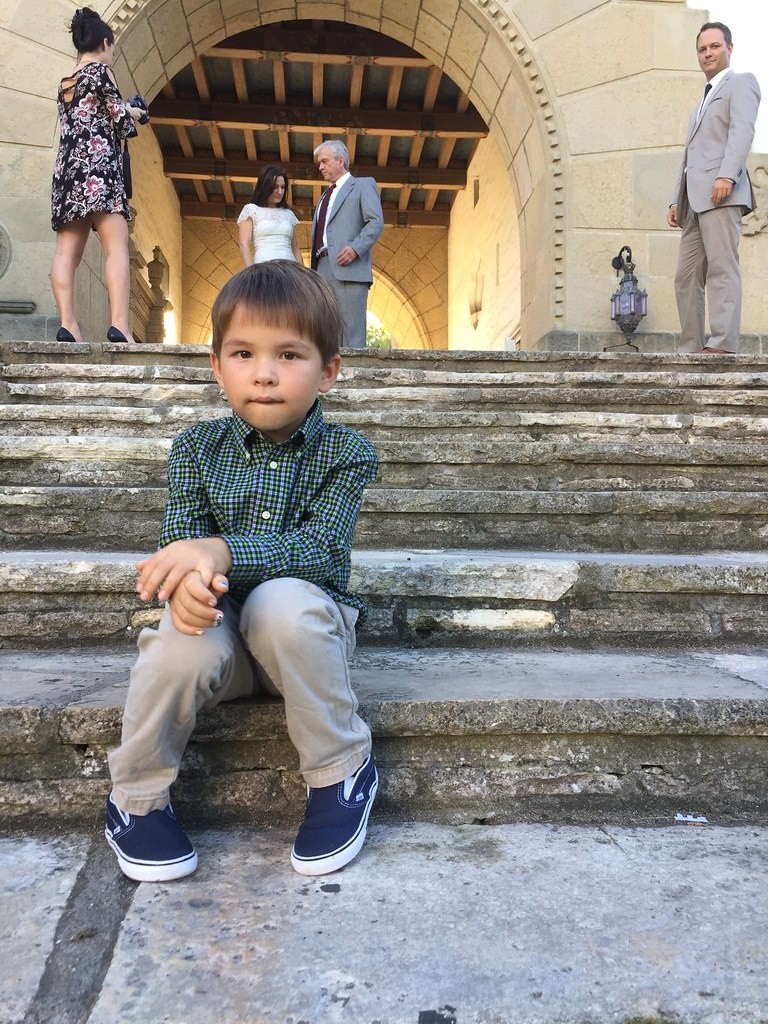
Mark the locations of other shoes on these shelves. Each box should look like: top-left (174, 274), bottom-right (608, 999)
top-left (56, 327), bottom-right (76, 342)
top-left (104, 789), bottom-right (198, 882)
top-left (702, 348), bottom-right (726, 353)
top-left (107, 326), bottom-right (128, 342)
top-left (290, 752), bottom-right (379, 876)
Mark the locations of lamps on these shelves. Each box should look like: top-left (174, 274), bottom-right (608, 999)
top-left (603, 246), bottom-right (648, 352)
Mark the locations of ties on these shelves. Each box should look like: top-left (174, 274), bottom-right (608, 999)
top-left (316, 184), bottom-right (337, 251)
top-left (700, 84), bottom-right (712, 114)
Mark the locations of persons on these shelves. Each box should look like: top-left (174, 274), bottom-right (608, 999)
top-left (311, 140), bottom-right (384, 349)
top-left (237, 163), bottom-right (304, 268)
top-left (667, 22), bottom-right (761, 354)
top-left (51, 7), bottom-right (149, 343)
top-left (103, 258), bottom-right (379, 880)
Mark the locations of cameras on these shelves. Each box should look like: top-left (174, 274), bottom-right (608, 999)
top-left (127, 95), bottom-right (150, 125)
